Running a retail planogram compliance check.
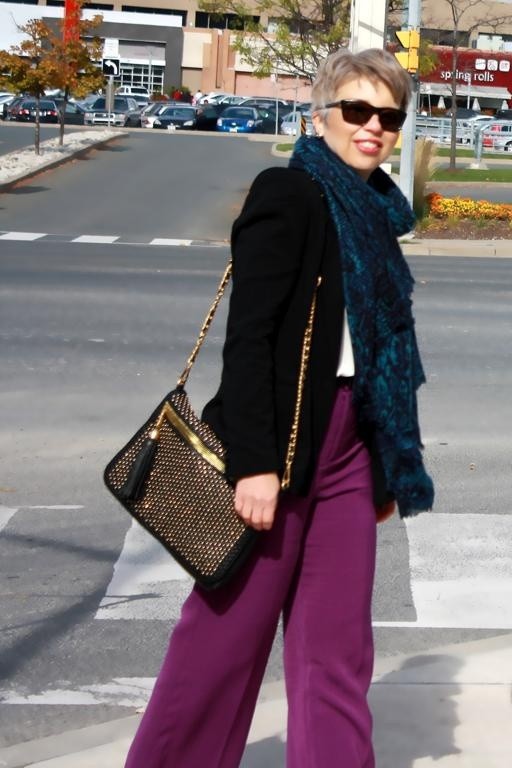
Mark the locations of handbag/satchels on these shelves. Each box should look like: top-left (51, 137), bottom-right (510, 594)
top-left (98, 385), bottom-right (292, 595)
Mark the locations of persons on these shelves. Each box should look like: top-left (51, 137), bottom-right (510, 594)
top-left (122, 50), bottom-right (435, 765)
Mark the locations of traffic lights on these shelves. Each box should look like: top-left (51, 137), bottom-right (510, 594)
top-left (393, 30), bottom-right (420, 71)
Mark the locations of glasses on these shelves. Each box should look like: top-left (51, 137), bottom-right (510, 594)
top-left (311, 95), bottom-right (408, 133)
